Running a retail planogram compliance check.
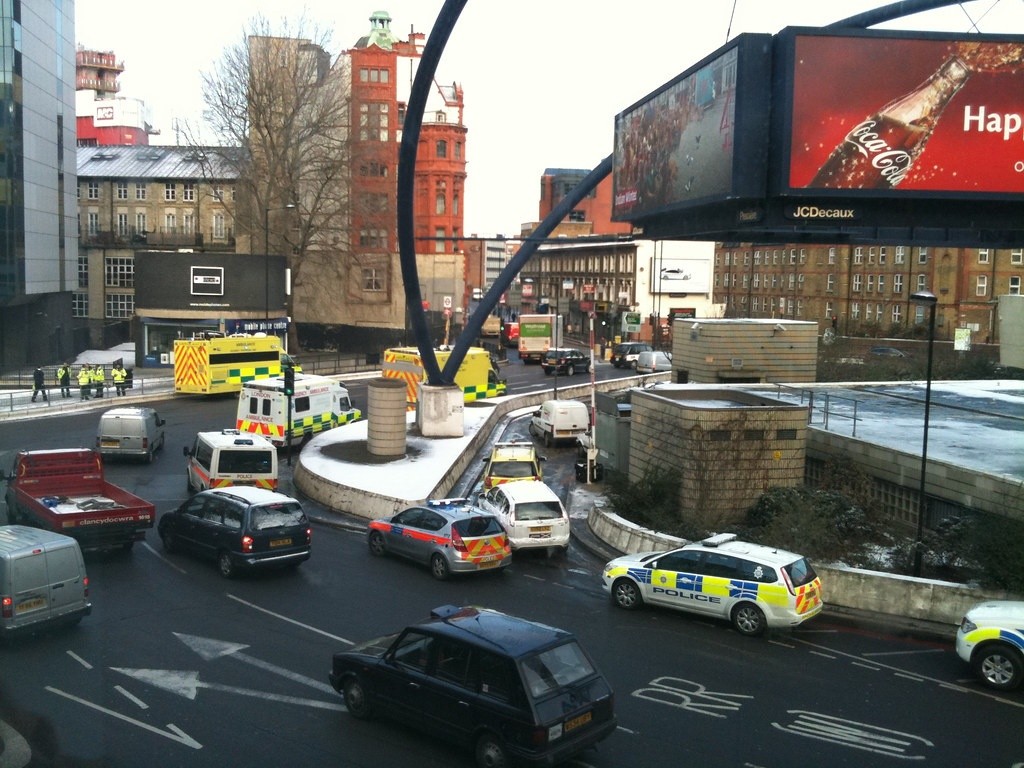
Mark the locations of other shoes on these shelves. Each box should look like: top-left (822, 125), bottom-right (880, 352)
top-left (44, 399), bottom-right (48, 401)
top-left (100, 396), bottom-right (103, 397)
top-left (94, 396), bottom-right (99, 398)
top-left (67, 395), bottom-right (73, 397)
top-left (31, 399), bottom-right (36, 402)
top-left (62, 395), bottom-right (65, 398)
top-left (85, 398), bottom-right (89, 400)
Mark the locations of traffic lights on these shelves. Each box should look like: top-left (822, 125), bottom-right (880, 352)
top-left (500, 319), bottom-right (503, 331)
top-left (283, 368), bottom-right (295, 396)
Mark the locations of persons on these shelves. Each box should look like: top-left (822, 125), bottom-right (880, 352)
top-left (76, 362), bottom-right (95, 401)
top-left (111, 364), bottom-right (127, 396)
top-left (30, 364), bottom-right (48, 402)
top-left (56, 361), bottom-right (72, 397)
top-left (94, 364), bottom-right (104, 398)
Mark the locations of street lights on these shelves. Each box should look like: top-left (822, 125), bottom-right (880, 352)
top-left (910, 291), bottom-right (938, 575)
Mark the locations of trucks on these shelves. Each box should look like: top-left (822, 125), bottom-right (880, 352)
top-left (383, 345), bottom-right (507, 411)
top-left (236, 373), bottom-right (361, 452)
top-left (173, 333), bottom-right (303, 395)
top-left (519, 314), bottom-right (564, 365)
top-left (0, 448), bottom-right (156, 561)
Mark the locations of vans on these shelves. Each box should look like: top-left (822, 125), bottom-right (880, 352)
top-left (183, 428), bottom-right (278, 493)
top-left (529, 399), bottom-right (590, 448)
top-left (481, 316), bottom-right (672, 377)
top-left (0, 524), bottom-right (93, 640)
top-left (96, 407), bottom-right (166, 463)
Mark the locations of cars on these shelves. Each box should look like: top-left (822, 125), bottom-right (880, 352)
top-left (481, 441), bottom-right (546, 494)
top-left (956, 601), bottom-right (1023, 691)
top-left (601, 533), bottom-right (826, 638)
top-left (478, 479), bottom-right (570, 557)
top-left (367, 498), bottom-right (513, 582)
top-left (328, 605), bottom-right (620, 768)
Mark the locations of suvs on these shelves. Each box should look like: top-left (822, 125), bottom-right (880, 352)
top-left (157, 487), bottom-right (312, 579)
